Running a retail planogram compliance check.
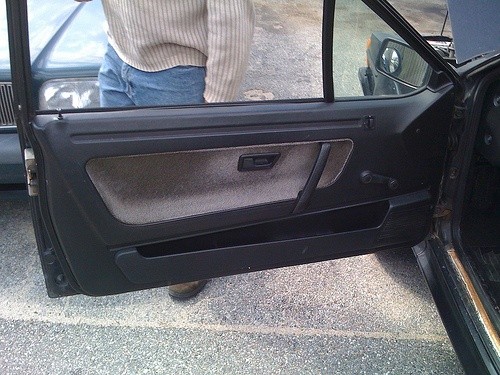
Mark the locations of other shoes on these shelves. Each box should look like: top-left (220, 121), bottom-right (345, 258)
top-left (168, 279), bottom-right (208, 298)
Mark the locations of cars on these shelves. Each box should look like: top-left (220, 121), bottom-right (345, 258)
top-left (0, 0), bottom-right (109, 187)
top-left (6, 0), bottom-right (500, 375)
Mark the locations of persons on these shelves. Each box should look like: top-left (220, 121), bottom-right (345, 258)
top-left (74, 0), bottom-right (257, 301)
